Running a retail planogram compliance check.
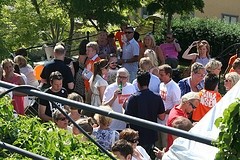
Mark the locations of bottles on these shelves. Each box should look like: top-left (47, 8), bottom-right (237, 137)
top-left (118, 81), bottom-right (122, 94)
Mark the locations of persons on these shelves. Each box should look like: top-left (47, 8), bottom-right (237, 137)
top-left (153, 33), bottom-right (240, 160)
top-left (80, 27), bottom-right (165, 132)
top-left (92, 106), bottom-right (120, 152)
top-left (124, 71), bottom-right (166, 160)
top-left (112, 140), bottom-right (134, 160)
top-left (38, 41), bottom-right (95, 142)
top-left (119, 128), bottom-right (152, 160)
top-left (0, 47), bottom-right (40, 116)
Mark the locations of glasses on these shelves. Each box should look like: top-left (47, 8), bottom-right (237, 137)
top-left (166, 37), bottom-right (172, 39)
top-left (117, 76), bottom-right (128, 79)
top-left (125, 32), bottom-right (133, 34)
top-left (126, 139), bottom-right (137, 143)
top-left (189, 102), bottom-right (196, 109)
top-left (224, 79), bottom-right (231, 83)
top-left (110, 62), bottom-right (117, 64)
top-left (56, 116), bottom-right (67, 121)
